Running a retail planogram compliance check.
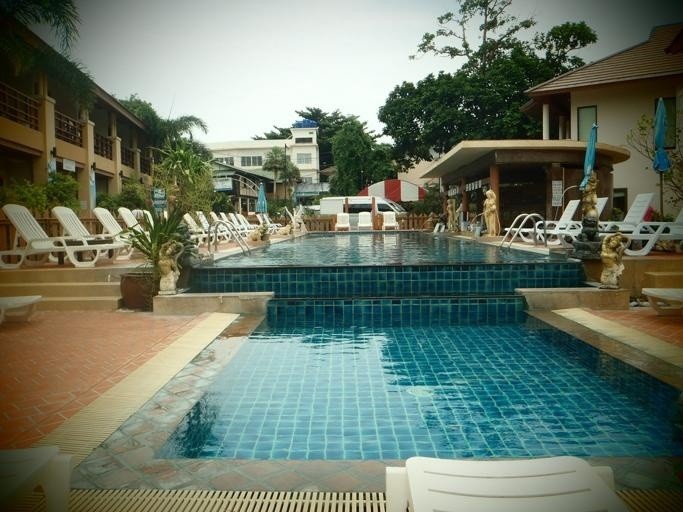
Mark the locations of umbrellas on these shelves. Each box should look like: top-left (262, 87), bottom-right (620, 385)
top-left (578, 121), bottom-right (597, 190)
top-left (651, 97), bottom-right (670, 221)
top-left (254, 183), bottom-right (267, 224)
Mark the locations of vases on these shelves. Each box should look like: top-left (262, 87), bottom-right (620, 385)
top-left (119, 272), bottom-right (159, 311)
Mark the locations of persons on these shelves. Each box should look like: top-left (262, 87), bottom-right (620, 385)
top-left (583, 171), bottom-right (599, 221)
top-left (599, 232), bottom-right (629, 285)
top-left (157, 240), bottom-right (185, 291)
top-left (481, 190), bottom-right (498, 236)
top-left (446, 199), bottom-right (456, 232)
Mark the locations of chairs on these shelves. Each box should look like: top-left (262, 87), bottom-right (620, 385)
top-left (334, 211), bottom-right (399, 231)
top-left (503, 192), bottom-right (682, 256)
top-left (0, 203), bottom-right (279, 269)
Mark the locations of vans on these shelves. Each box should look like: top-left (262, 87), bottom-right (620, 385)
top-left (319, 196), bottom-right (408, 228)
